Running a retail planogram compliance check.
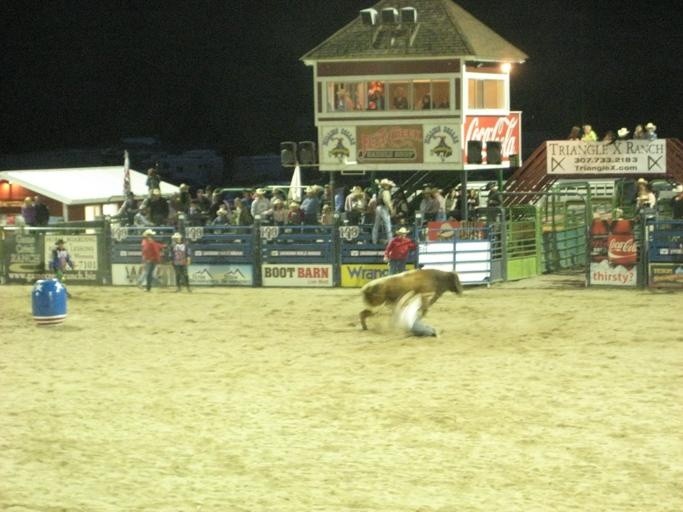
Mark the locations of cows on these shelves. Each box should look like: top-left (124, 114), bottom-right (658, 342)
top-left (357, 269), bottom-right (463, 335)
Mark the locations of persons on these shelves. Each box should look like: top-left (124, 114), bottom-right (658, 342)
top-left (616, 127), bottom-right (630, 140)
top-left (383, 227), bottom-right (417, 275)
top-left (48, 236), bottom-right (77, 299)
top-left (589, 176), bottom-right (683, 221)
top-left (135, 229), bottom-right (163, 291)
top-left (632, 124), bottom-right (642, 138)
top-left (20, 197), bottom-right (38, 233)
top-left (642, 122), bottom-right (657, 140)
top-left (567, 126), bottom-right (580, 141)
top-left (33, 196), bottom-right (49, 227)
top-left (111, 169), bottom-right (502, 244)
top-left (335, 86), bottom-right (449, 111)
top-left (579, 124), bottom-right (597, 141)
top-left (601, 131), bottom-right (615, 142)
top-left (167, 232), bottom-right (192, 294)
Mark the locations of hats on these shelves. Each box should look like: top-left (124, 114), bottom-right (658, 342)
top-left (151, 189), bottom-right (161, 195)
top-left (178, 183), bottom-right (191, 190)
top-left (395, 227), bottom-right (411, 234)
top-left (173, 232), bottom-right (182, 238)
top-left (422, 186), bottom-right (438, 193)
top-left (55, 240), bottom-right (65, 245)
top-left (634, 178), bottom-right (649, 186)
top-left (251, 185), bottom-right (333, 212)
top-left (143, 230), bottom-right (157, 236)
top-left (350, 186), bottom-right (365, 195)
top-left (618, 128), bottom-right (630, 137)
top-left (672, 185), bottom-right (683, 192)
top-left (645, 122), bottom-right (657, 131)
top-left (378, 179), bottom-right (396, 188)
top-left (217, 208), bottom-right (227, 215)
top-left (636, 195), bottom-right (649, 199)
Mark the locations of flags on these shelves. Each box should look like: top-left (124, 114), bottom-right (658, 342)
top-left (121, 156), bottom-right (132, 193)
top-left (288, 159), bottom-right (301, 202)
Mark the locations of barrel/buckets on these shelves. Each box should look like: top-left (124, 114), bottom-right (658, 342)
top-left (590, 217), bottom-right (605, 261)
top-left (32, 279), bottom-right (67, 325)
top-left (608, 219), bottom-right (637, 265)
